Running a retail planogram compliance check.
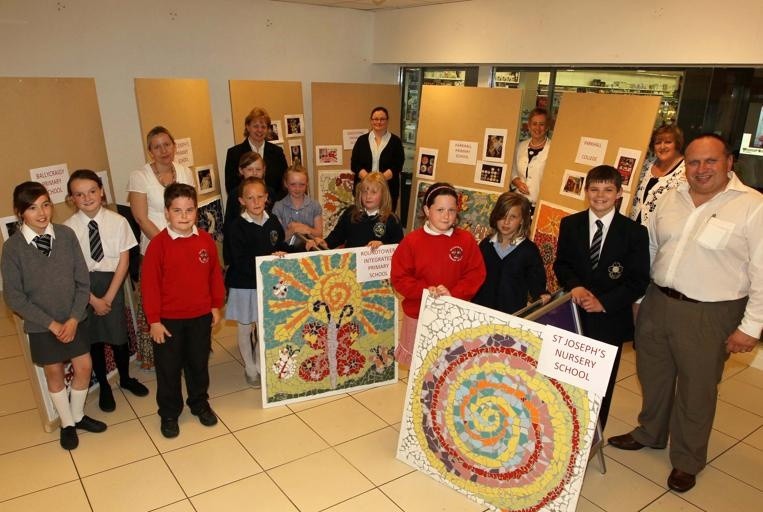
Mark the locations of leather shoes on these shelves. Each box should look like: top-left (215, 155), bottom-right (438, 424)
top-left (607, 428), bottom-right (668, 454)
top-left (666, 467), bottom-right (699, 494)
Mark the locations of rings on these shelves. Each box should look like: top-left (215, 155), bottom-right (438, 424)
top-left (740, 350), bottom-right (746, 354)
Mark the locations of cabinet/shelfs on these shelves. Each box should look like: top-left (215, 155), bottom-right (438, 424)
top-left (403, 69), bottom-right (676, 144)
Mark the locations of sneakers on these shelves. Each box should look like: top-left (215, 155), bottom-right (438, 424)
top-left (191, 404), bottom-right (219, 427)
top-left (58, 426), bottom-right (80, 450)
top-left (244, 362), bottom-right (262, 389)
top-left (159, 415), bottom-right (181, 439)
top-left (97, 383), bottom-right (117, 411)
top-left (119, 377), bottom-right (151, 398)
top-left (74, 415), bottom-right (106, 433)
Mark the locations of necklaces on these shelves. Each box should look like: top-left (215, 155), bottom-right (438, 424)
top-left (149, 159), bottom-right (176, 189)
top-left (529, 137), bottom-right (547, 148)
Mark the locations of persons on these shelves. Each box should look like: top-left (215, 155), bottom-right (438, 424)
top-left (631, 123), bottom-right (688, 353)
top-left (222, 107), bottom-right (289, 206)
top-left (0, 181), bottom-right (107, 449)
top-left (605, 129), bottom-right (762, 494)
top-left (138, 183), bottom-right (226, 439)
top-left (389, 182), bottom-right (487, 387)
top-left (222, 174), bottom-right (288, 390)
top-left (270, 160), bottom-right (324, 252)
top-left (311, 171), bottom-right (404, 255)
top-left (123, 125), bottom-right (197, 375)
top-left (473, 189), bottom-right (554, 316)
top-left (348, 106), bottom-right (406, 217)
top-left (551, 164), bottom-right (652, 436)
top-left (59, 168), bottom-right (149, 414)
top-left (221, 151), bottom-right (280, 359)
top-left (506, 106), bottom-right (552, 220)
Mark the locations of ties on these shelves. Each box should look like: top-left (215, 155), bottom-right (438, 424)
top-left (589, 219), bottom-right (604, 273)
top-left (32, 233), bottom-right (52, 257)
top-left (87, 220), bottom-right (104, 264)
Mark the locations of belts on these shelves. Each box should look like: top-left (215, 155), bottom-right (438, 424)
top-left (651, 281), bottom-right (711, 303)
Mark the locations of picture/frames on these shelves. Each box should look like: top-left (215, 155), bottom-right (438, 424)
top-left (739, 97), bottom-right (763, 157)
